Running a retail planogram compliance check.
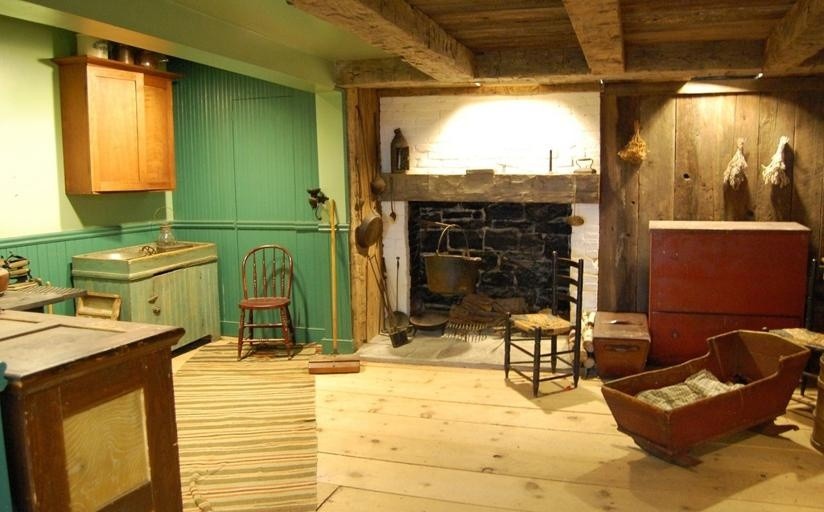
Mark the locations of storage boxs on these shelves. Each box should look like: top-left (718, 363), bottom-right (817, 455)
top-left (648, 221), bottom-right (811, 370)
top-left (594, 312), bottom-right (650, 380)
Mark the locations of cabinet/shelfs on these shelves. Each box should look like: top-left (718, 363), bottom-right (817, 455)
top-left (73, 263), bottom-right (221, 358)
top-left (50, 55), bottom-right (183, 194)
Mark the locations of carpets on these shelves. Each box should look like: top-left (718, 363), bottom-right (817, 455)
top-left (174, 337), bottom-right (321, 512)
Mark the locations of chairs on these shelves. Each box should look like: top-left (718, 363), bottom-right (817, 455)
top-left (238, 244), bottom-right (296, 360)
top-left (502, 249), bottom-right (583, 398)
